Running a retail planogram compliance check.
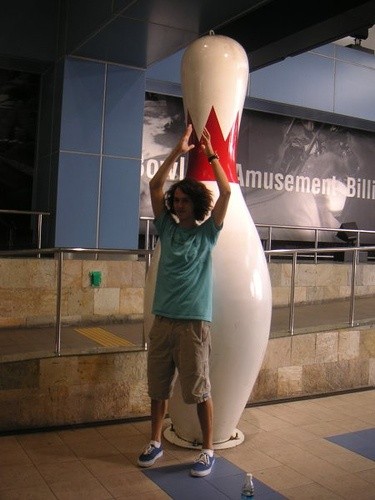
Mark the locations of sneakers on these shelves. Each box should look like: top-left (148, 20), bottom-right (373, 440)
top-left (190, 452), bottom-right (215, 477)
top-left (135, 443), bottom-right (164, 467)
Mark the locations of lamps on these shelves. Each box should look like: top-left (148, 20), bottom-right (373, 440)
top-left (336, 221), bottom-right (363, 247)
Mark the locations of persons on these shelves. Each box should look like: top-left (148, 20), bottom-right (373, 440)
top-left (138, 124), bottom-right (231, 477)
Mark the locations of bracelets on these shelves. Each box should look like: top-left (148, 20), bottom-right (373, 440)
top-left (207, 152), bottom-right (219, 164)
